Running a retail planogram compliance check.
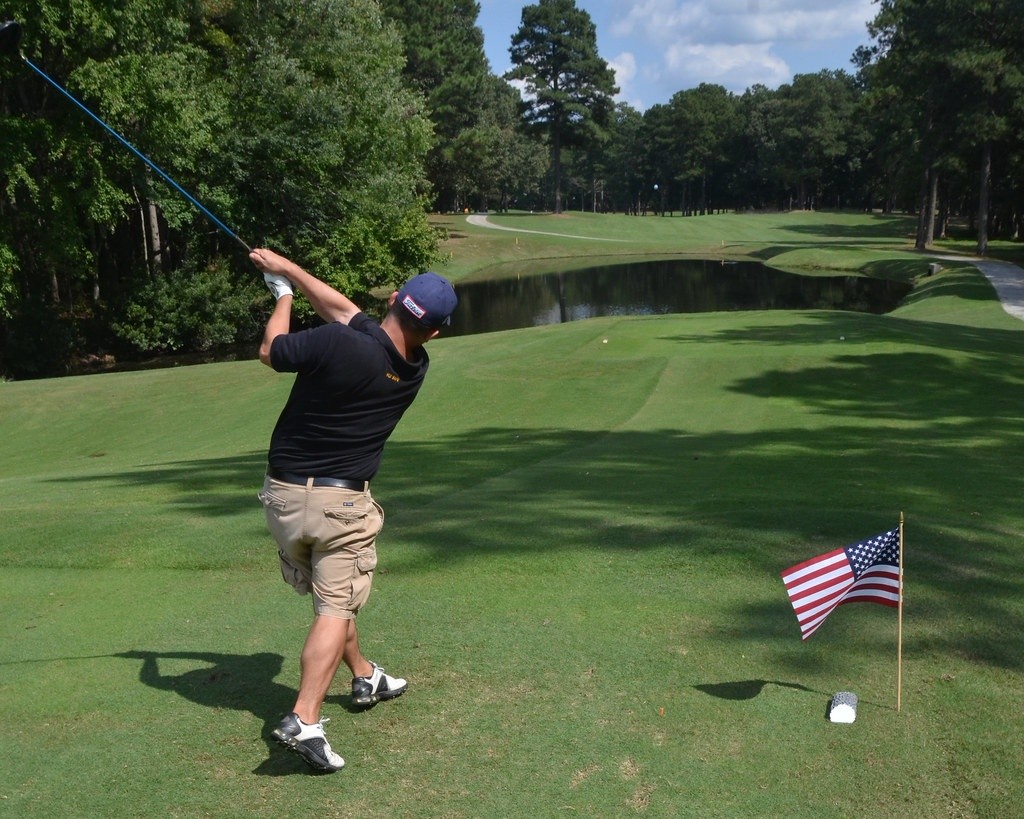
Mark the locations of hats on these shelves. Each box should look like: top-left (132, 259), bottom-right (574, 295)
top-left (396, 273), bottom-right (457, 328)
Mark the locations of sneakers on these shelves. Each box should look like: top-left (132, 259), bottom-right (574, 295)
top-left (350, 660), bottom-right (409, 708)
top-left (270, 712), bottom-right (346, 772)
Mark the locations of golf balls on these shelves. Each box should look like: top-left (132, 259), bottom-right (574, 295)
top-left (653, 183), bottom-right (659, 190)
top-left (602, 339), bottom-right (608, 344)
top-left (840, 336), bottom-right (845, 341)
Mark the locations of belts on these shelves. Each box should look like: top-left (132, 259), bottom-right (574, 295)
top-left (267, 464), bottom-right (369, 492)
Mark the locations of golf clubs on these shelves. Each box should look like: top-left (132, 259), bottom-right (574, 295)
top-left (0, 20), bottom-right (256, 255)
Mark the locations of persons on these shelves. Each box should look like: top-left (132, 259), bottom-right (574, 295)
top-left (245, 249), bottom-right (460, 772)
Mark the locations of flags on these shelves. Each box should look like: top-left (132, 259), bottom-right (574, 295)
top-left (781, 526), bottom-right (903, 640)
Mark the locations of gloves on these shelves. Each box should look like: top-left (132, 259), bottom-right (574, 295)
top-left (262, 272), bottom-right (296, 299)
